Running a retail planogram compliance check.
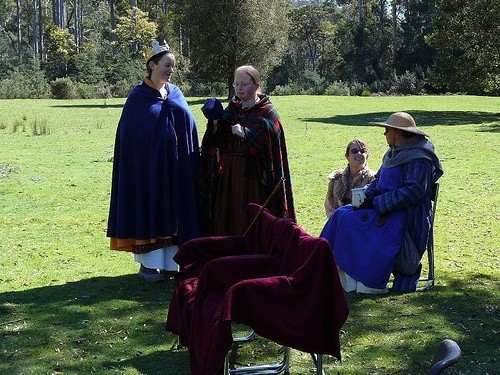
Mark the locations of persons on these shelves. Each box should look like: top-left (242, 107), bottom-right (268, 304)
top-left (106, 41), bottom-right (198, 281)
top-left (319, 112), bottom-right (444, 294)
top-left (199, 66), bottom-right (299, 238)
top-left (325, 138), bottom-right (377, 219)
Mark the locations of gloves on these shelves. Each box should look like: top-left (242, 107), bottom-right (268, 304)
top-left (360, 197), bottom-right (374, 209)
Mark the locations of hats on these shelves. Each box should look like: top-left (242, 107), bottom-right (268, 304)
top-left (368, 112), bottom-right (430, 137)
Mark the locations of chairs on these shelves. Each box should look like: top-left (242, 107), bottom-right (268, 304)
top-left (372, 182), bottom-right (438, 291)
top-left (427, 339), bottom-right (463, 375)
top-left (166, 203), bottom-right (350, 375)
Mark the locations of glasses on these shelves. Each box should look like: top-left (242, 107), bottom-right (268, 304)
top-left (350, 148), bottom-right (367, 154)
top-left (232, 82), bottom-right (256, 87)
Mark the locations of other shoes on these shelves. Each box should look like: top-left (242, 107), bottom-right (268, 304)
top-left (138, 264), bottom-right (165, 283)
